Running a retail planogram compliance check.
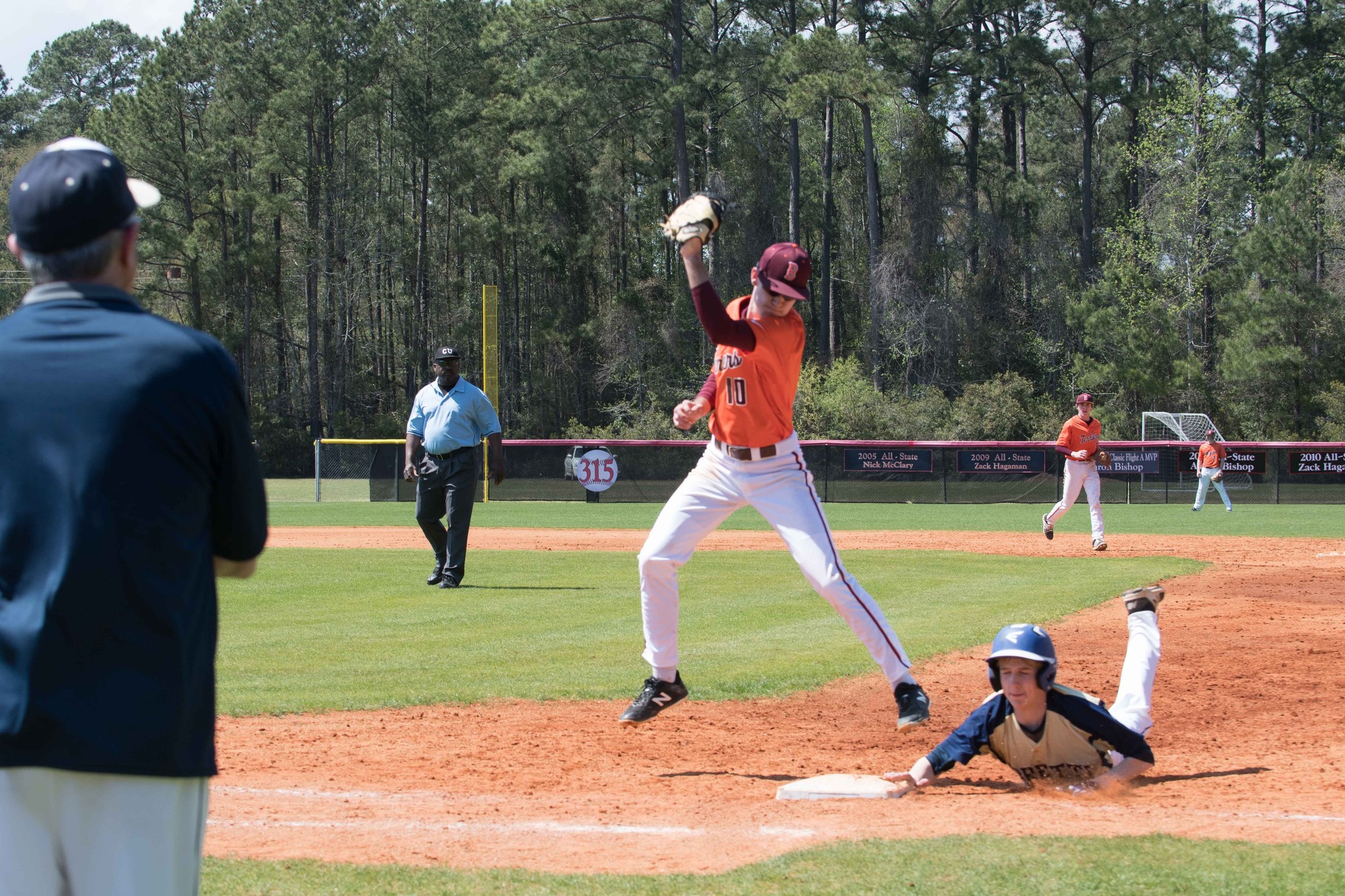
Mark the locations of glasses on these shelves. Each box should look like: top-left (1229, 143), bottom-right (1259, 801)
top-left (435, 360), bottom-right (459, 367)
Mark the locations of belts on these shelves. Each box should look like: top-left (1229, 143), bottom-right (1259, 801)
top-left (1075, 458), bottom-right (1092, 462)
top-left (425, 447), bottom-right (473, 460)
top-left (715, 437), bottom-right (776, 462)
top-left (1206, 467), bottom-right (1218, 469)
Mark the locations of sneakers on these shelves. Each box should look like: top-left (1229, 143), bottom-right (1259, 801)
top-left (1042, 514), bottom-right (1053, 540)
top-left (427, 567), bottom-right (443, 585)
top-left (438, 573), bottom-right (458, 588)
top-left (618, 669), bottom-right (689, 727)
top-left (1121, 584), bottom-right (1165, 616)
top-left (894, 682), bottom-right (931, 731)
top-left (1092, 538), bottom-right (1108, 551)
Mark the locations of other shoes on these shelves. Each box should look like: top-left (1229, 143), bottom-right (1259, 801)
top-left (1191, 507), bottom-right (1198, 511)
top-left (1226, 510), bottom-right (1231, 512)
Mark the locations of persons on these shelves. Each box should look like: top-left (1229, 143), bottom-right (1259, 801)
top-left (403, 347), bottom-right (509, 591)
top-left (0, 136), bottom-right (268, 896)
top-left (884, 585), bottom-right (1165, 798)
top-left (1042, 394), bottom-right (1112, 551)
top-left (619, 193), bottom-right (930, 732)
top-left (1191, 430), bottom-right (1233, 512)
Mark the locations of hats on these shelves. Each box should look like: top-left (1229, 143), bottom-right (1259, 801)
top-left (1205, 430), bottom-right (1214, 436)
top-left (759, 242), bottom-right (811, 300)
top-left (434, 346), bottom-right (460, 363)
top-left (9, 136), bottom-right (160, 255)
top-left (1076, 393), bottom-right (1094, 405)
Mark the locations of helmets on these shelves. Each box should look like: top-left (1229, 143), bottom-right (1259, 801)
top-left (985, 623), bottom-right (1057, 693)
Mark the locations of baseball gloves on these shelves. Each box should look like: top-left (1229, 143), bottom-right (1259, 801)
top-left (1210, 471), bottom-right (1223, 483)
top-left (661, 191), bottom-right (727, 249)
top-left (1092, 448), bottom-right (1111, 469)
